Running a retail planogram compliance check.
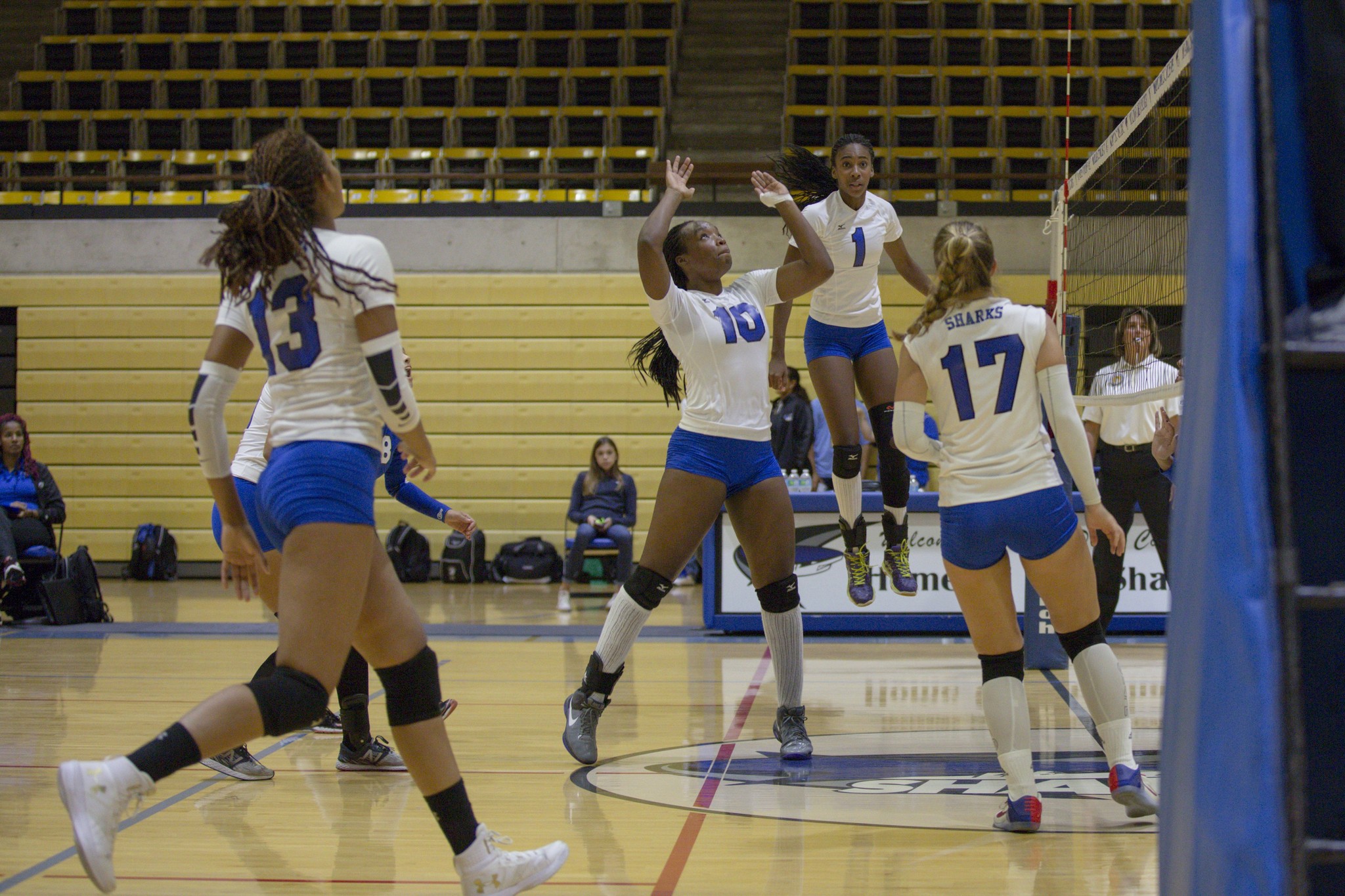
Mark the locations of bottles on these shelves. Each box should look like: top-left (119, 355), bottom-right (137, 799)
top-left (781, 468), bottom-right (789, 481)
top-left (909, 474), bottom-right (919, 492)
top-left (799, 469), bottom-right (813, 492)
top-left (787, 469), bottom-right (799, 493)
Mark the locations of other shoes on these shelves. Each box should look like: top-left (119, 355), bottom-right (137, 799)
top-left (556, 586), bottom-right (571, 611)
top-left (605, 592), bottom-right (619, 610)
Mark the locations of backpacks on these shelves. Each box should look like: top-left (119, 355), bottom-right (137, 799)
top-left (439, 526), bottom-right (486, 585)
top-left (126, 578), bottom-right (178, 622)
top-left (442, 584), bottom-right (485, 625)
top-left (40, 544), bottom-right (114, 624)
top-left (119, 522), bottom-right (178, 583)
top-left (385, 518), bottom-right (431, 584)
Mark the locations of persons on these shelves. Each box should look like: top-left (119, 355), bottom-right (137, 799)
top-left (767, 366), bottom-right (940, 493)
top-left (336, 341), bottom-right (478, 772)
top-left (199, 378), bottom-right (343, 781)
top-left (892, 220), bottom-right (1159, 833)
top-left (58, 128), bottom-right (569, 896)
top-left (562, 154), bottom-right (834, 763)
top-left (0, 413), bottom-right (66, 624)
top-left (766, 135), bottom-right (935, 606)
top-left (1075, 307), bottom-right (1187, 639)
top-left (557, 437), bottom-right (637, 611)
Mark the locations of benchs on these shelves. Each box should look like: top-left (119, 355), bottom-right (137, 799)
top-left (0, 274), bottom-right (939, 562)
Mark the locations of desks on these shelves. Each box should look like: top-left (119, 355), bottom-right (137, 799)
top-left (702, 492), bottom-right (1172, 633)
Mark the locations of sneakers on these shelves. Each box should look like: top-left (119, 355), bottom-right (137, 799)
top-left (292, 706), bottom-right (343, 733)
top-left (200, 742), bottom-right (275, 780)
top-left (772, 705), bottom-right (813, 760)
top-left (881, 539), bottom-right (918, 597)
top-left (0, 579), bottom-right (13, 603)
top-left (2, 555), bottom-right (28, 595)
top-left (56, 758), bottom-right (157, 895)
top-left (335, 769), bottom-right (414, 803)
top-left (195, 779), bottom-right (275, 825)
top-left (842, 540), bottom-right (879, 607)
top-left (335, 735), bottom-right (409, 771)
top-left (1108, 763), bottom-right (1159, 818)
top-left (452, 821), bottom-right (569, 896)
top-left (562, 766), bottom-right (610, 837)
top-left (562, 686), bottom-right (612, 763)
top-left (992, 791), bottom-right (1042, 831)
top-left (773, 758), bottom-right (813, 812)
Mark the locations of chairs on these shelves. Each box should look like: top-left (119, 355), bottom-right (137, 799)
top-left (562, 514), bottom-right (633, 593)
top-left (0, 0), bottom-right (1189, 190)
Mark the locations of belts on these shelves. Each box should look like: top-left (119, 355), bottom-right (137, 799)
top-left (1101, 441), bottom-right (1152, 452)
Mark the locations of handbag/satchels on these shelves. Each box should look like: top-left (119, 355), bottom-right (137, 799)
top-left (488, 536), bottom-right (563, 584)
top-left (671, 554), bottom-right (700, 586)
